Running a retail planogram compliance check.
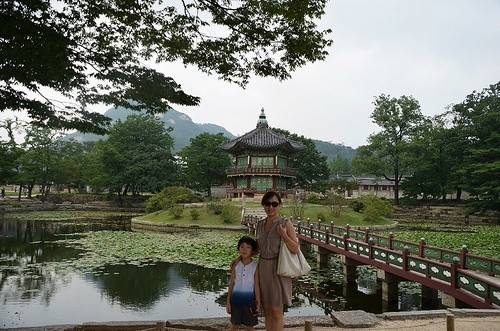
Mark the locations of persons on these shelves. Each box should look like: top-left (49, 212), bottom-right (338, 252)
top-left (226, 236), bottom-right (261, 331)
top-left (230, 192), bottom-right (299, 331)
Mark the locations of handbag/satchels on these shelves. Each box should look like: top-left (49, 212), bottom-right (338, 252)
top-left (276, 218), bottom-right (312, 278)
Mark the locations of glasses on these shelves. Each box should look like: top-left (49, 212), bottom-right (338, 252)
top-left (264, 200), bottom-right (280, 207)
